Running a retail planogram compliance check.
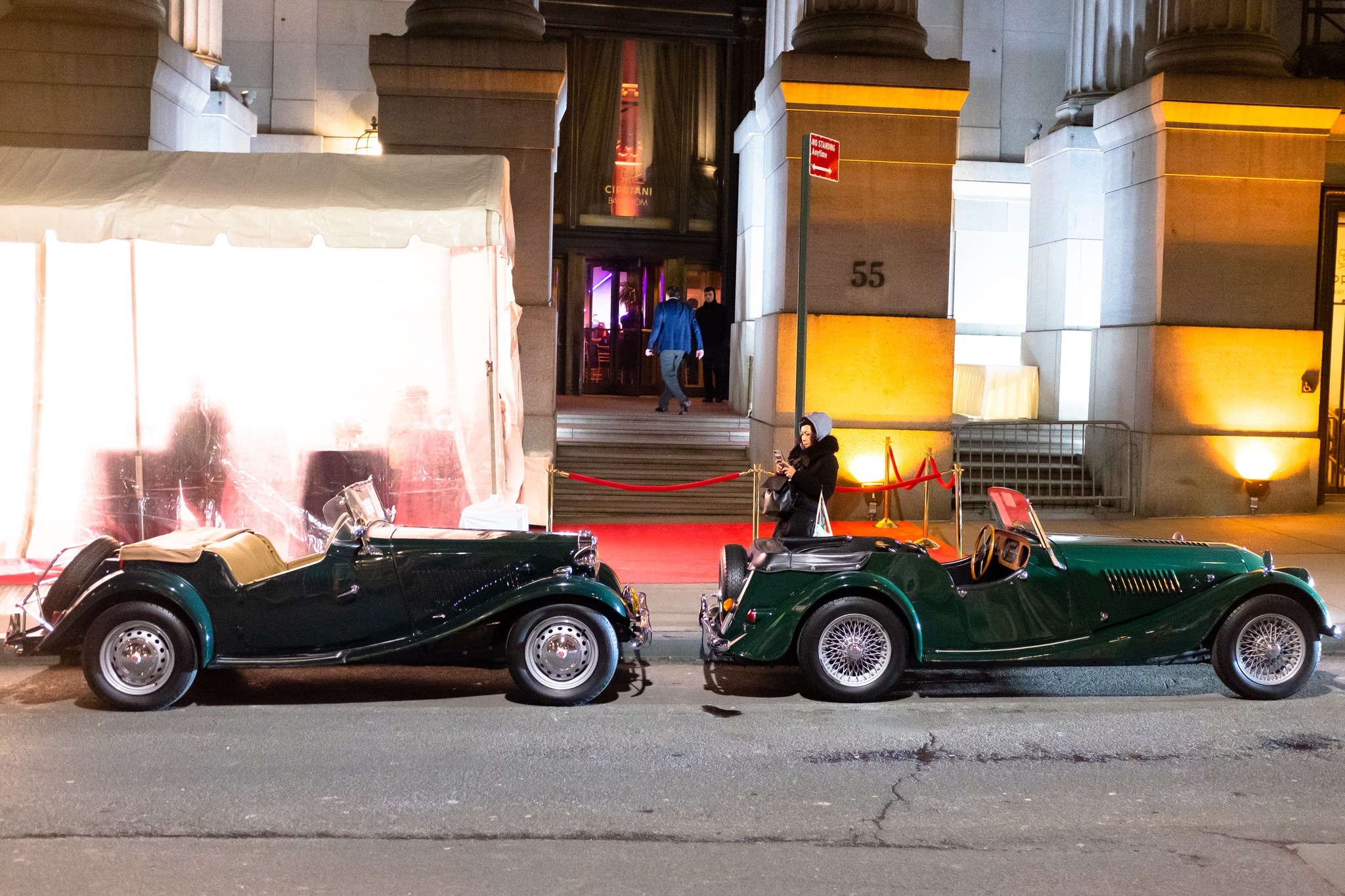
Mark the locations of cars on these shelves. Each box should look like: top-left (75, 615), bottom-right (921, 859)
top-left (0, 476), bottom-right (653, 712)
top-left (697, 484), bottom-right (1341, 704)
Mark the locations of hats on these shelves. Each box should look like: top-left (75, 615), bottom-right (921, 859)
top-left (800, 417), bottom-right (811, 426)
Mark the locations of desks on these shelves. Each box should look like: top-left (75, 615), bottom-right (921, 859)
top-left (94, 449), bottom-right (186, 544)
top-left (299, 451), bottom-right (400, 528)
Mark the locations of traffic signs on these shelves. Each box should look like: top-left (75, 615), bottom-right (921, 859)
top-left (809, 131), bottom-right (841, 183)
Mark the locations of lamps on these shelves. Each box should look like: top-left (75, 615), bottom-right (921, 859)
top-left (1246, 479), bottom-right (1269, 497)
top-left (241, 90), bottom-right (249, 109)
top-left (1068, 97), bottom-right (1081, 125)
top-left (1030, 119), bottom-right (1042, 140)
top-left (354, 116), bottom-right (381, 155)
top-left (862, 483), bottom-right (885, 521)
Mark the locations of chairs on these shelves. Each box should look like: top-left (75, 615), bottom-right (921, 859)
top-left (584, 341), bottom-right (622, 384)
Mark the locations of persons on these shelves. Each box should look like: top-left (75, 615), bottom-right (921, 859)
top-left (585, 322), bottom-right (610, 367)
top-left (619, 302), bottom-right (644, 384)
top-left (592, 314), bottom-right (601, 328)
top-left (772, 412), bottom-right (839, 537)
top-left (696, 286), bottom-right (732, 402)
top-left (645, 286), bottom-right (704, 415)
top-left (687, 298), bottom-right (698, 385)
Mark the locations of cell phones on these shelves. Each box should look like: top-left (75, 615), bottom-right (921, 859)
top-left (774, 450), bottom-right (787, 469)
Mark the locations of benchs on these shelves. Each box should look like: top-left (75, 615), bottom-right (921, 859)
top-left (206, 531), bottom-right (288, 586)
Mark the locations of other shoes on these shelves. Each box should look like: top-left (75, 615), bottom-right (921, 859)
top-left (679, 400), bottom-right (691, 414)
top-left (655, 407), bottom-right (669, 413)
top-left (702, 397), bottom-right (713, 402)
top-left (715, 399), bottom-right (722, 402)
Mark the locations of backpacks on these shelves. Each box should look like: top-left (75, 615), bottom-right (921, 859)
top-left (760, 461), bottom-right (802, 516)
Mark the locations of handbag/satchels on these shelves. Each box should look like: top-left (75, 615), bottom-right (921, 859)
top-left (812, 492), bottom-right (832, 537)
